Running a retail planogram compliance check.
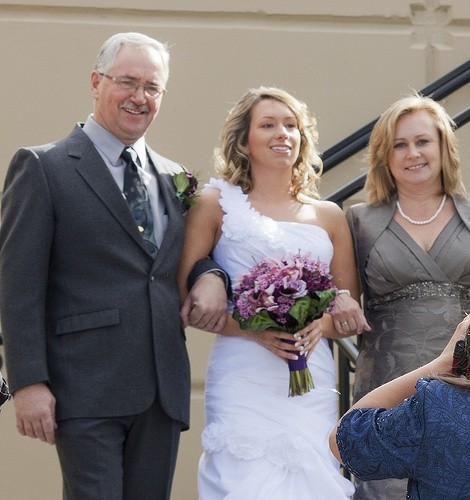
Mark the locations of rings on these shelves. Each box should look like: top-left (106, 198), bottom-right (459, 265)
top-left (341, 321), bottom-right (348, 326)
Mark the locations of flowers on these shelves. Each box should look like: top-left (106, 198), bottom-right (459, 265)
top-left (231, 249), bottom-right (337, 400)
top-left (173, 170), bottom-right (199, 217)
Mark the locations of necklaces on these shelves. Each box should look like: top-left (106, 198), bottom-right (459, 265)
top-left (396, 193), bottom-right (446, 225)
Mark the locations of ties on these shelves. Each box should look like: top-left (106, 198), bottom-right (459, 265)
top-left (121, 146), bottom-right (157, 258)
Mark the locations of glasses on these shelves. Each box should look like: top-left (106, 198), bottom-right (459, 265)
top-left (97, 71), bottom-right (166, 101)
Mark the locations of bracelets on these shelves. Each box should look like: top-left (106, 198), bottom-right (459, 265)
top-left (337, 290), bottom-right (351, 296)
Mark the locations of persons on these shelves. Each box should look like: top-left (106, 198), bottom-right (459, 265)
top-left (0, 32), bottom-right (234, 500)
top-left (179, 86), bottom-right (361, 500)
top-left (329, 313), bottom-right (470, 500)
top-left (330, 88), bottom-right (470, 500)
